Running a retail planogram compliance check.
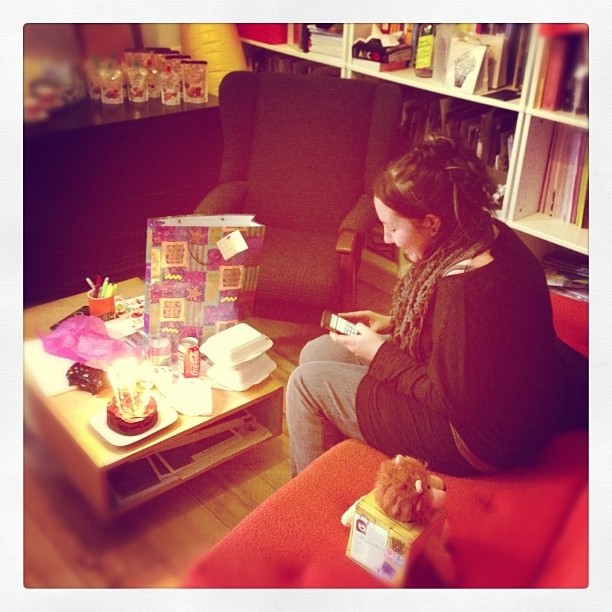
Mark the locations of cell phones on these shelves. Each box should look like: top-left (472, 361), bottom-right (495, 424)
top-left (320, 311), bottom-right (361, 336)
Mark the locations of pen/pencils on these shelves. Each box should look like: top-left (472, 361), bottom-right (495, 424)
top-left (86, 276), bottom-right (118, 298)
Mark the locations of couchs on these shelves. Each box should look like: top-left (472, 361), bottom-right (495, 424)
top-left (171, 294), bottom-right (589, 587)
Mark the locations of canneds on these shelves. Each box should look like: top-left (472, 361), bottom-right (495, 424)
top-left (179, 337), bottom-right (200, 378)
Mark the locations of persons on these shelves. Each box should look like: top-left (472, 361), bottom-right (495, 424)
top-left (285, 133), bottom-right (555, 483)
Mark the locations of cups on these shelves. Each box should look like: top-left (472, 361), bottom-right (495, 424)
top-left (87, 287), bottom-right (125, 320)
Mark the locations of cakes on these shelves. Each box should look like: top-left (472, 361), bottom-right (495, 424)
top-left (104, 391), bottom-right (157, 435)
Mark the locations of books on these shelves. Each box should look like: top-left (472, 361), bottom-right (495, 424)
top-left (308, 25), bottom-right (345, 61)
top-left (541, 247), bottom-right (589, 300)
top-left (537, 121), bottom-right (589, 229)
top-left (456, 24), bottom-right (532, 91)
top-left (532, 27), bottom-right (589, 124)
top-left (381, 24), bottom-right (415, 45)
top-left (351, 38), bottom-right (414, 81)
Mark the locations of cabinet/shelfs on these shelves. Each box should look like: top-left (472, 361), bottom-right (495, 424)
top-left (237, 24), bottom-right (591, 304)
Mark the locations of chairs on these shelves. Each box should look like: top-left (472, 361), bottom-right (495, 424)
top-left (54, 92), bottom-right (222, 283)
top-left (190, 70), bottom-right (405, 326)
